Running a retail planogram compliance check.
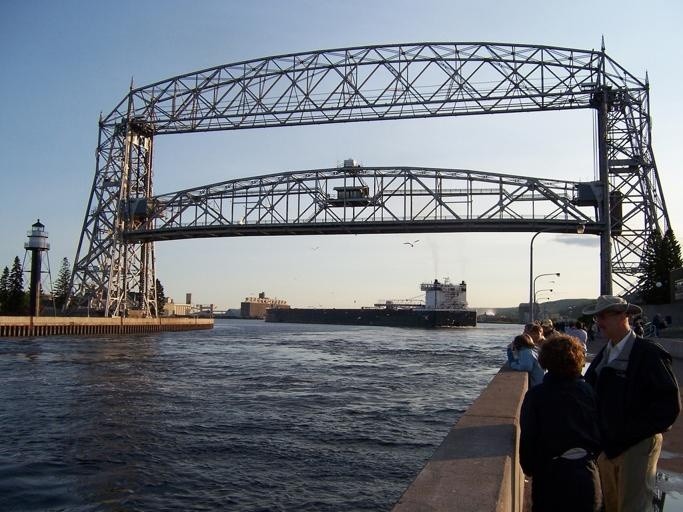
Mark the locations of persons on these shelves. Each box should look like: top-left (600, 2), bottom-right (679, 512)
top-left (507, 313), bottom-right (661, 386)
top-left (582, 295), bottom-right (680, 512)
top-left (519, 335), bottom-right (603, 512)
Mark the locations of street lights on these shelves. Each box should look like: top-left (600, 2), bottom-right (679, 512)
top-left (529, 224), bottom-right (585, 322)
top-left (534, 273), bottom-right (560, 320)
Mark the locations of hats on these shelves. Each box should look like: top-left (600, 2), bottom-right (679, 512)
top-left (582, 295), bottom-right (643, 314)
top-left (542, 319), bottom-right (553, 327)
